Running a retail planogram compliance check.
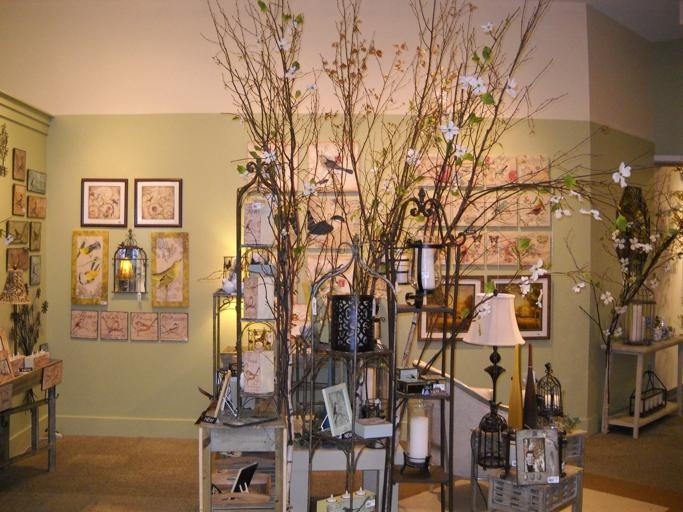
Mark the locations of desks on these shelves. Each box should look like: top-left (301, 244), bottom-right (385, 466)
top-left (2, 359), bottom-right (64, 474)
top-left (290, 440), bottom-right (406, 512)
top-left (395, 390), bottom-right (454, 512)
top-left (488, 463), bottom-right (586, 512)
top-left (195, 406), bottom-right (289, 512)
top-left (468, 423), bottom-right (590, 511)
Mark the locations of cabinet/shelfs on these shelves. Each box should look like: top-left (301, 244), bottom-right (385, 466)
top-left (597, 333), bottom-right (682, 442)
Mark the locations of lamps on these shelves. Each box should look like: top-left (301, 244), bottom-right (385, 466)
top-left (460, 290), bottom-right (530, 434)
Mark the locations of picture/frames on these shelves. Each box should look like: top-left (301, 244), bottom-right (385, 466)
top-left (321, 383), bottom-right (353, 440)
top-left (515, 428), bottom-right (563, 489)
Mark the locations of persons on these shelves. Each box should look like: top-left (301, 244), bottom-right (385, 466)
top-left (524, 450), bottom-right (543, 473)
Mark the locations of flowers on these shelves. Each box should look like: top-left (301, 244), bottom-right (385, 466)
top-left (201, 1), bottom-right (683, 512)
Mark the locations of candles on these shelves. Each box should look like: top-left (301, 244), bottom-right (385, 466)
top-left (410, 417), bottom-right (430, 463)
